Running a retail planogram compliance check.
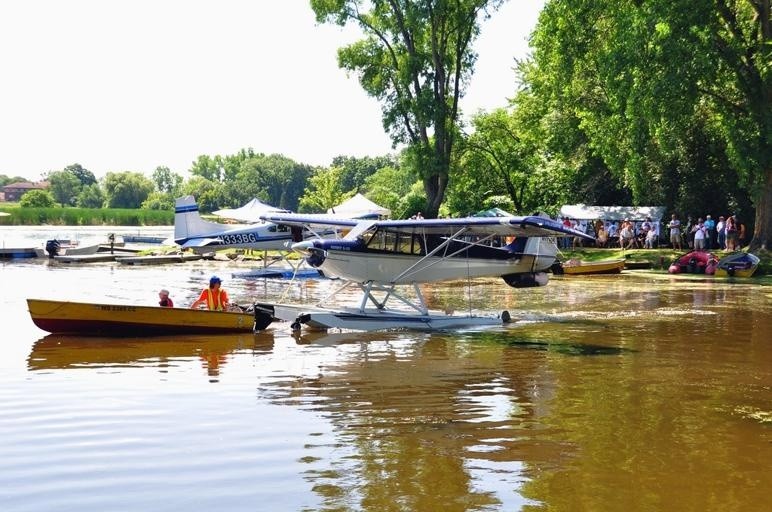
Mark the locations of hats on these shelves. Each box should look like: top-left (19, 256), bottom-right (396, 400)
top-left (210, 278), bottom-right (222, 283)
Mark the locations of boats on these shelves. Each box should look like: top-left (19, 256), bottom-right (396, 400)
top-left (44, 238), bottom-right (80, 248)
top-left (667, 248), bottom-right (718, 276)
top-left (0, 245), bottom-right (38, 258)
top-left (45, 240), bottom-right (101, 259)
top-left (25, 331), bottom-right (275, 365)
top-left (715, 248), bottom-right (762, 277)
top-left (23, 296), bottom-right (279, 336)
top-left (561, 258), bottom-right (627, 274)
top-left (120, 232), bottom-right (167, 243)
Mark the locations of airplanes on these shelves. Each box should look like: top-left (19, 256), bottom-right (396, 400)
top-left (243, 211), bottom-right (600, 341)
top-left (170, 193), bottom-right (383, 281)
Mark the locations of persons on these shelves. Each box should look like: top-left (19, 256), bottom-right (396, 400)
top-left (561, 213), bottom-right (747, 252)
top-left (159, 289), bottom-right (173, 307)
top-left (192, 277), bottom-right (229, 311)
